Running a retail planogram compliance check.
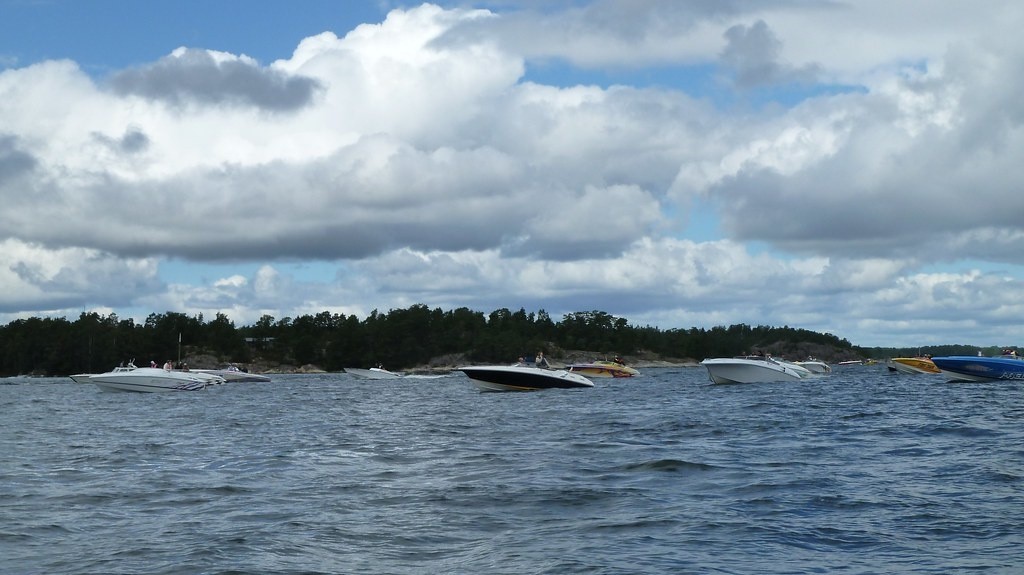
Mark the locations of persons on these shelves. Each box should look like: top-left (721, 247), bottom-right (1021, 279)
top-left (535, 351), bottom-right (551, 370)
top-left (1001, 348), bottom-right (1021, 360)
top-left (613, 355), bottom-right (626, 365)
top-left (519, 357), bottom-right (529, 365)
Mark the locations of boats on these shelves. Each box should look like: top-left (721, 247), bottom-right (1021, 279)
top-left (930, 355), bottom-right (1024, 380)
top-left (565, 358), bottom-right (640, 379)
top-left (188, 367), bottom-right (272, 384)
top-left (457, 364), bottom-right (596, 390)
top-left (767, 357), bottom-right (812, 379)
top-left (698, 354), bottom-right (803, 382)
top-left (839, 359), bottom-right (863, 365)
top-left (343, 363), bottom-right (402, 381)
top-left (865, 359), bottom-right (878, 365)
top-left (69, 372), bottom-right (97, 383)
top-left (87, 359), bottom-right (227, 392)
top-left (890, 354), bottom-right (942, 377)
top-left (790, 360), bottom-right (832, 373)
